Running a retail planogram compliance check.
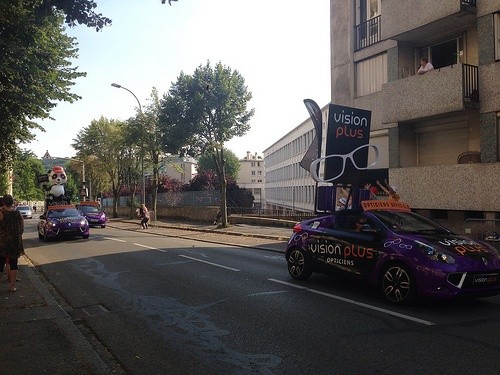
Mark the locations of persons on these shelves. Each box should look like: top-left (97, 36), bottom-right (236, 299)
top-left (34, 203), bottom-right (37, 213)
top-left (336, 179), bottom-right (401, 234)
top-left (0, 193), bottom-right (25, 292)
top-left (417, 57), bottom-right (433, 74)
top-left (137, 204), bottom-right (150, 229)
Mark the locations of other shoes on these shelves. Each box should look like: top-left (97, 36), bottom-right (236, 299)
top-left (16, 278), bottom-right (21, 281)
top-left (143, 227), bottom-right (145, 229)
top-left (146, 227), bottom-right (148, 229)
top-left (10, 288), bottom-right (16, 291)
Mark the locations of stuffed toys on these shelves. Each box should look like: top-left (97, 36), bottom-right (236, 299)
top-left (42, 166), bottom-right (68, 202)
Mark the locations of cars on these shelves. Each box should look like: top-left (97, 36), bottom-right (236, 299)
top-left (78, 205), bottom-right (106, 227)
top-left (37, 206), bottom-right (89, 238)
top-left (286, 199), bottom-right (499, 314)
top-left (16, 206), bottom-right (31, 218)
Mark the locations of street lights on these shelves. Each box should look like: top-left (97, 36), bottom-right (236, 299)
top-left (111, 82), bottom-right (145, 206)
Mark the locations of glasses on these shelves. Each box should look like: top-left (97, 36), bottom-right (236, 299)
top-left (310, 144), bottom-right (380, 182)
